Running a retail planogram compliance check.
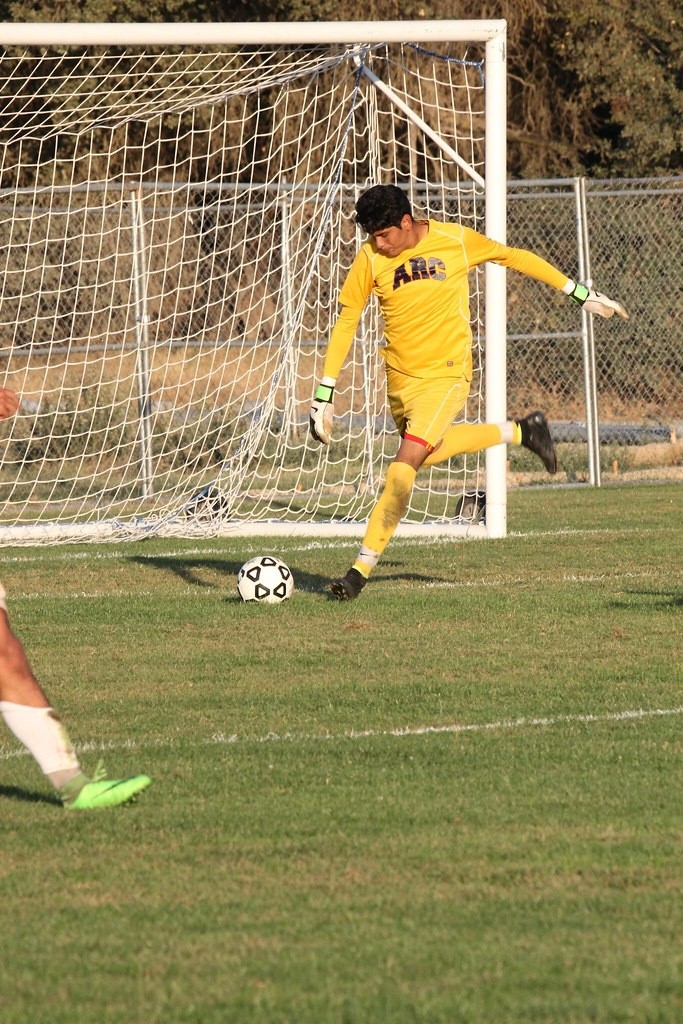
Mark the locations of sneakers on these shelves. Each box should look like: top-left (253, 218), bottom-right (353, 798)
top-left (329, 569), bottom-right (366, 600)
top-left (519, 410), bottom-right (557, 476)
top-left (57, 771), bottom-right (152, 809)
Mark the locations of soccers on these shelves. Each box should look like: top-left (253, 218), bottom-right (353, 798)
top-left (235, 556), bottom-right (295, 604)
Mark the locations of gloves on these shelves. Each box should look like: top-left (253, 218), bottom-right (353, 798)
top-left (309, 375), bottom-right (336, 445)
top-left (567, 284), bottom-right (630, 321)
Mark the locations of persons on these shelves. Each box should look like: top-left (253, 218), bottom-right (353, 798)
top-left (0, 389), bottom-right (152, 810)
top-left (309, 184), bottom-right (630, 601)
